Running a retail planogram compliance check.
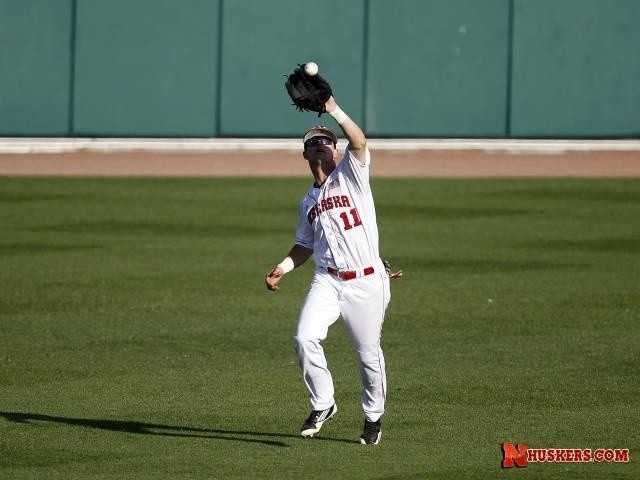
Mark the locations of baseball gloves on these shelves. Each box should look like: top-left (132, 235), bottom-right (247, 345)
top-left (285, 63), bottom-right (333, 113)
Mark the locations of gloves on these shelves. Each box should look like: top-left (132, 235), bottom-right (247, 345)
top-left (389, 270), bottom-right (402, 280)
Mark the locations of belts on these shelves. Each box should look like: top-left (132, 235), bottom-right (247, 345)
top-left (327, 267), bottom-right (374, 280)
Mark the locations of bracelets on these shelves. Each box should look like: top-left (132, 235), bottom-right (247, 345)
top-left (330, 104), bottom-right (349, 126)
top-left (276, 256), bottom-right (295, 276)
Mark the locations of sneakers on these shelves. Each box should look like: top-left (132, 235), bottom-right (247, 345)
top-left (300, 401), bottom-right (337, 437)
top-left (361, 419), bottom-right (382, 445)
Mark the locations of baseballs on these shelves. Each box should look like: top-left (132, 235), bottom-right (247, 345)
top-left (304, 62), bottom-right (319, 75)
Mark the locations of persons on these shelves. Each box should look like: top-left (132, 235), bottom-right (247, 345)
top-left (262, 63), bottom-right (403, 444)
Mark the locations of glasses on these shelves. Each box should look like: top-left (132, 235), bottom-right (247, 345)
top-left (306, 138), bottom-right (333, 147)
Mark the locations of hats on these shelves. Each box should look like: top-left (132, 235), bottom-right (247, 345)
top-left (303, 125), bottom-right (338, 149)
